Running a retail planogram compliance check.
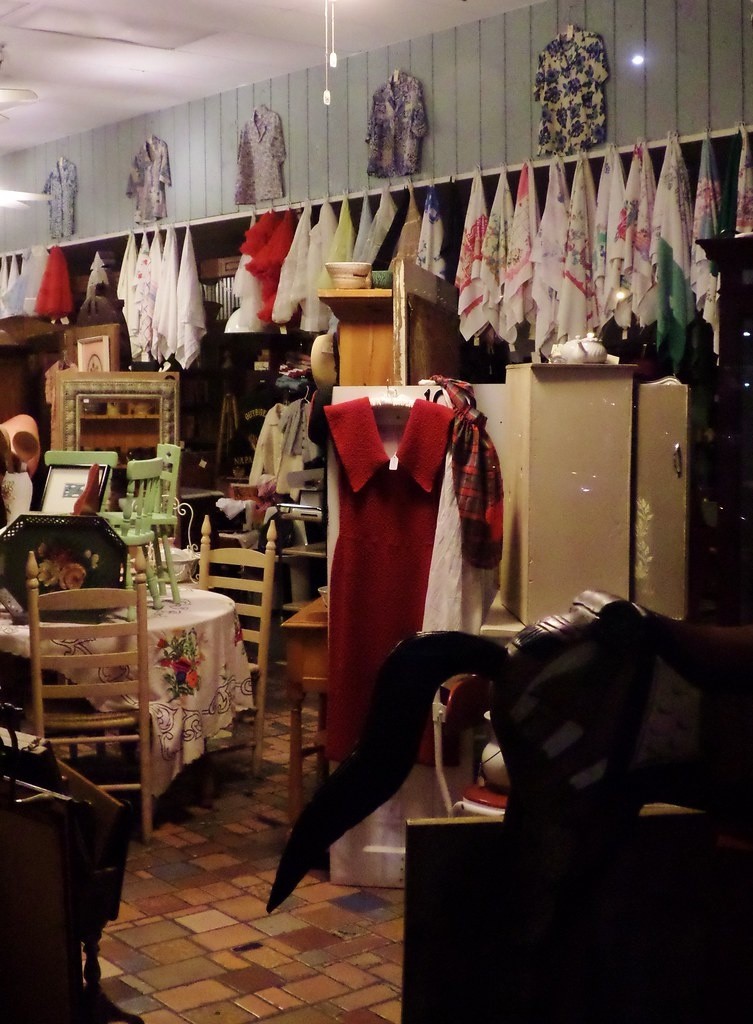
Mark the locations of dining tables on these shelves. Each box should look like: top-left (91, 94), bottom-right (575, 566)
top-left (0, 583), bottom-right (253, 810)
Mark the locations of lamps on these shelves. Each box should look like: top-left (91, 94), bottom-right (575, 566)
top-left (0, 89), bottom-right (39, 103)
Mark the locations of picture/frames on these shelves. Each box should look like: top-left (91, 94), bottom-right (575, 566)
top-left (60, 378), bottom-right (178, 542)
top-left (39, 463), bottom-right (111, 513)
top-left (77, 335), bottom-right (110, 373)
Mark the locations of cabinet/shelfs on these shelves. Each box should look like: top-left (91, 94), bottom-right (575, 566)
top-left (498, 361), bottom-right (689, 629)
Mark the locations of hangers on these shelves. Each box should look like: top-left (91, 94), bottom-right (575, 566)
top-left (368, 376), bottom-right (415, 408)
top-left (301, 384), bottom-right (312, 404)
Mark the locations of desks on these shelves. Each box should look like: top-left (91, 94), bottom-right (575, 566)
top-left (279, 596), bottom-right (328, 828)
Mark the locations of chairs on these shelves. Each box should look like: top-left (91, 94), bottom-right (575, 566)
top-left (0, 546), bottom-right (153, 845)
top-left (96, 443), bottom-right (181, 605)
top-left (432, 673), bottom-right (510, 817)
top-left (110, 456), bottom-right (164, 622)
top-left (44, 449), bottom-right (118, 516)
top-left (198, 512), bottom-right (276, 778)
